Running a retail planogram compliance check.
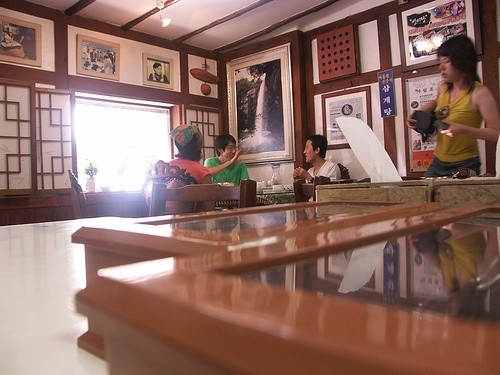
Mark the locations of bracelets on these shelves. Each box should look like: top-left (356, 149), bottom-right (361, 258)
top-left (307, 177), bottom-right (314, 184)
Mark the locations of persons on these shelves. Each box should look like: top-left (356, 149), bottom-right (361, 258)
top-left (82, 46), bottom-right (116, 75)
top-left (204, 134), bottom-right (249, 185)
top-left (149, 63), bottom-right (169, 84)
top-left (406, 34), bottom-right (500, 179)
top-left (292, 134), bottom-right (341, 202)
top-left (164, 124), bottom-right (214, 213)
top-left (436, 222), bottom-right (500, 320)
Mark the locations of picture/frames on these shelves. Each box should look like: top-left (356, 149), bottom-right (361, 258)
top-left (142, 52), bottom-right (174, 90)
top-left (227, 42), bottom-right (296, 165)
top-left (76, 33), bottom-right (120, 81)
top-left (321, 85), bottom-right (372, 151)
top-left (402, 67), bottom-right (447, 178)
top-left (394, 0), bottom-right (483, 74)
top-left (0, 14), bottom-right (42, 67)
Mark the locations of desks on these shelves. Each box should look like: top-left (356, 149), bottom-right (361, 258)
top-left (208, 181), bottom-right (313, 206)
top-left (0, 202), bottom-right (500, 375)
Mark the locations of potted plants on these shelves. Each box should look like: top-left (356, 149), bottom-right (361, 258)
top-left (84, 163), bottom-right (98, 193)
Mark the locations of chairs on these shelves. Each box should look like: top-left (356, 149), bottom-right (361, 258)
top-left (292, 176), bottom-right (330, 204)
top-left (139, 160), bottom-right (204, 213)
top-left (149, 179), bottom-right (257, 218)
top-left (335, 162), bottom-right (351, 180)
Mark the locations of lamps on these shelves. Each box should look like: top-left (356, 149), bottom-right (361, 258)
top-left (156, 0), bottom-right (172, 29)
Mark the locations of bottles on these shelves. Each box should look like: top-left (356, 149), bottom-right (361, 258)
top-left (271, 163), bottom-right (282, 185)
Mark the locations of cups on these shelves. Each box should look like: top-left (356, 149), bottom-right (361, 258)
top-left (272, 185), bottom-right (282, 193)
